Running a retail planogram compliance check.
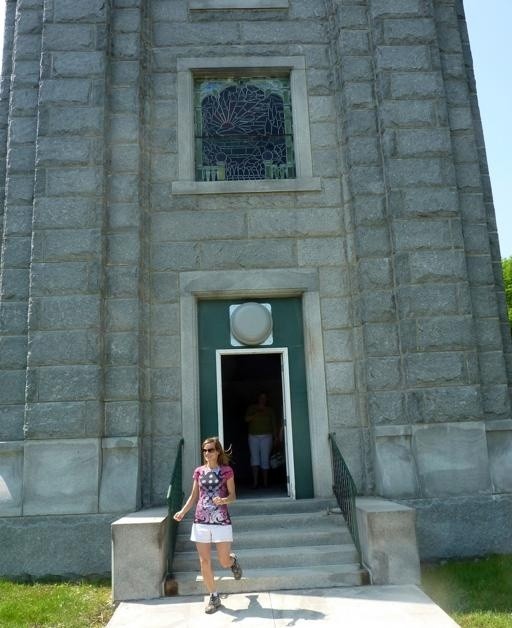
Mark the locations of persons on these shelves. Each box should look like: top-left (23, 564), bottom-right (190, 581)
top-left (173, 437), bottom-right (243, 613)
top-left (245, 391), bottom-right (277, 487)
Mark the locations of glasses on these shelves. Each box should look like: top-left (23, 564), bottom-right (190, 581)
top-left (202, 449), bottom-right (216, 453)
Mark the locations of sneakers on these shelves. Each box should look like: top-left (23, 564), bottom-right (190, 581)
top-left (230, 553), bottom-right (242, 579)
top-left (205, 593), bottom-right (221, 613)
